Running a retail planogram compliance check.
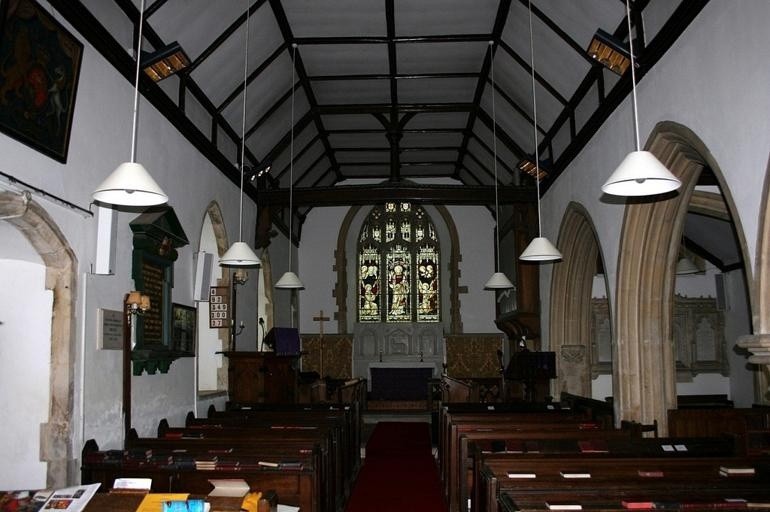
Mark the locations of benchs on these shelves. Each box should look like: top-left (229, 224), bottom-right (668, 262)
top-left (434, 401), bottom-right (770, 512)
top-left (1, 400), bottom-right (355, 512)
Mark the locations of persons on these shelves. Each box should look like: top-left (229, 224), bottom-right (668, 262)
top-left (389, 266), bottom-right (410, 316)
top-left (419, 283), bottom-right (437, 314)
top-left (361, 284), bottom-right (379, 315)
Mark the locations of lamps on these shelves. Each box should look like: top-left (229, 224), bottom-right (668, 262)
top-left (600, 0), bottom-right (683, 200)
top-left (219, 0), bottom-right (302, 289)
top-left (484, 1), bottom-right (562, 289)
top-left (94, 0), bottom-right (168, 209)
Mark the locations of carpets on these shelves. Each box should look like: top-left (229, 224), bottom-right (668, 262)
top-left (345, 420), bottom-right (451, 512)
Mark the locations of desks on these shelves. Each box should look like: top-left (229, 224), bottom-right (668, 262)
top-left (218, 349), bottom-right (310, 404)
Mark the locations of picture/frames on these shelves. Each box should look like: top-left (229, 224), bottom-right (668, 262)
top-left (171, 302), bottom-right (197, 359)
